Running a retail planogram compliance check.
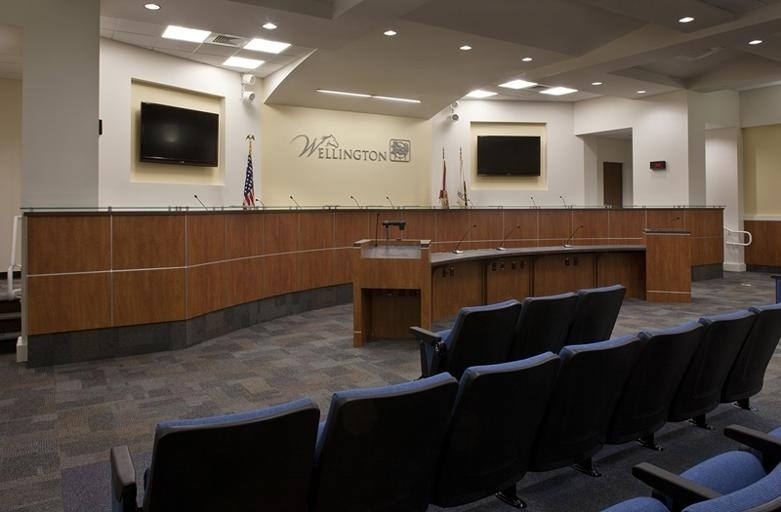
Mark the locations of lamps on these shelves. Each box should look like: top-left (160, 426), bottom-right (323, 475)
top-left (450, 99), bottom-right (461, 122)
top-left (240, 71), bottom-right (257, 104)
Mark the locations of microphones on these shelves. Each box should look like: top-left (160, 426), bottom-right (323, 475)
top-left (255, 198), bottom-right (266, 209)
top-left (350, 195), bottom-right (359, 206)
top-left (386, 197), bottom-right (393, 206)
top-left (496, 225), bottom-right (521, 250)
top-left (565, 225), bottom-right (584, 248)
top-left (559, 195), bottom-right (566, 207)
top-left (530, 196), bottom-right (538, 207)
top-left (289, 195), bottom-right (301, 207)
top-left (194, 194), bottom-right (209, 211)
top-left (655, 216), bottom-right (680, 229)
top-left (452, 223), bottom-right (476, 254)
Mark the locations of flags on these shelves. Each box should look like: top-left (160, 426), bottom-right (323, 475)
top-left (243, 151), bottom-right (256, 208)
top-left (439, 160), bottom-right (449, 206)
top-left (454, 162), bottom-right (471, 206)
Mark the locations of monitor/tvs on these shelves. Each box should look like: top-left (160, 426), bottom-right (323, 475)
top-left (477, 135), bottom-right (540, 176)
top-left (139, 101), bottom-right (219, 167)
